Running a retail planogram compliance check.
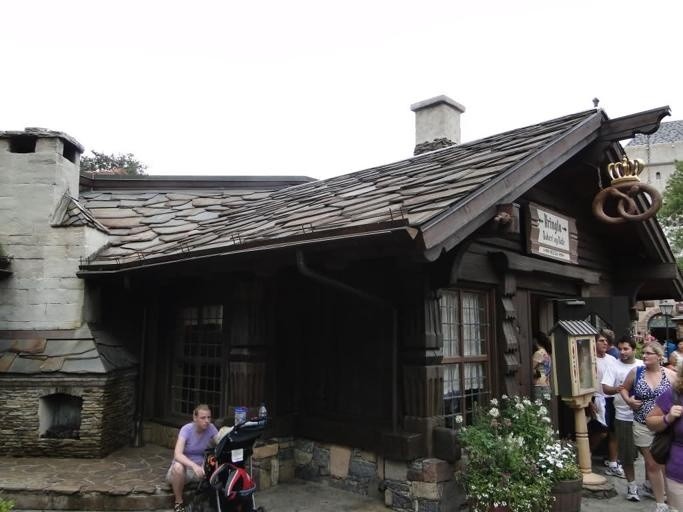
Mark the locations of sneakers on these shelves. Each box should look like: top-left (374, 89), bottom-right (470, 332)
top-left (625, 485), bottom-right (640, 501)
top-left (656, 503), bottom-right (667, 512)
top-left (604, 467), bottom-right (626, 479)
top-left (173, 503), bottom-right (186, 512)
top-left (641, 484), bottom-right (667, 502)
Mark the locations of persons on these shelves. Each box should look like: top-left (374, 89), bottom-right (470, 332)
top-left (590, 328), bottom-right (683, 512)
top-left (164, 403), bottom-right (219, 511)
top-left (529, 328), bottom-right (553, 412)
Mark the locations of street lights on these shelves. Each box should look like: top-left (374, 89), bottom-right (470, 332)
top-left (656, 298), bottom-right (674, 363)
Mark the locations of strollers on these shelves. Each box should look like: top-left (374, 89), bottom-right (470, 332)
top-left (181, 406), bottom-right (274, 512)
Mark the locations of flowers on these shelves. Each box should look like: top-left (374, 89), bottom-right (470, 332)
top-left (453, 391), bottom-right (582, 512)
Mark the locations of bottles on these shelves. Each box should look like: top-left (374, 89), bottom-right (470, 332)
top-left (233, 407), bottom-right (247, 425)
top-left (258, 403), bottom-right (268, 419)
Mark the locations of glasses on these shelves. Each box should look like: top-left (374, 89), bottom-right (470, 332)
top-left (641, 352), bottom-right (656, 355)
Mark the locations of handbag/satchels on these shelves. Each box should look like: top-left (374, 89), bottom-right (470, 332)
top-left (648, 431), bottom-right (671, 465)
